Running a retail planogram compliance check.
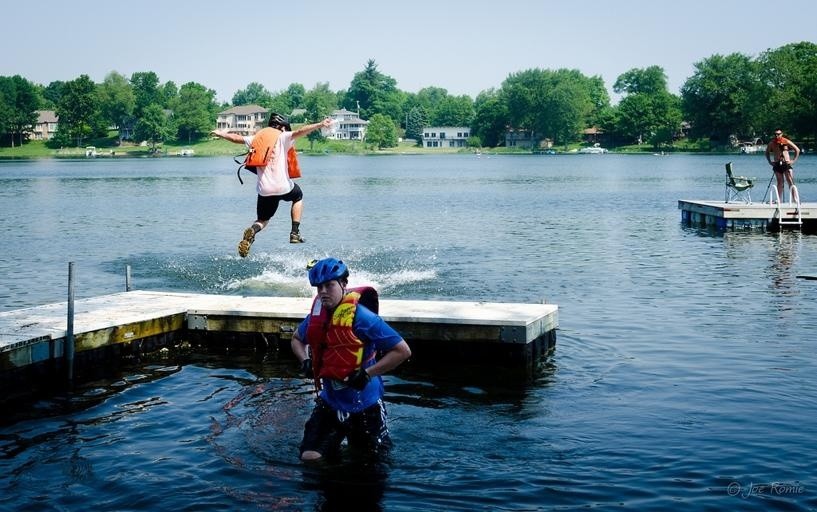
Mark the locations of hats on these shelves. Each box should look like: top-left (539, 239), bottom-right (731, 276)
top-left (269, 112), bottom-right (292, 131)
top-left (309, 258), bottom-right (349, 286)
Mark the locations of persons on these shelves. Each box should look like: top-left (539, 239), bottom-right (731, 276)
top-left (764, 129), bottom-right (801, 204)
top-left (209, 110), bottom-right (331, 257)
top-left (286, 258), bottom-right (411, 461)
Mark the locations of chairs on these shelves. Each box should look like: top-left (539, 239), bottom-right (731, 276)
top-left (723, 160), bottom-right (755, 204)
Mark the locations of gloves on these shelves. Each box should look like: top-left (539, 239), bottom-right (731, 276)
top-left (303, 359), bottom-right (314, 379)
top-left (344, 369), bottom-right (372, 390)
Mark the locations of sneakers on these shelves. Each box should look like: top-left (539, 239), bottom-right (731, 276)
top-left (238, 227), bottom-right (255, 257)
top-left (290, 232), bottom-right (306, 243)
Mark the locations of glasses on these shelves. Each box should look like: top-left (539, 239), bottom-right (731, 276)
top-left (775, 133), bottom-right (782, 135)
